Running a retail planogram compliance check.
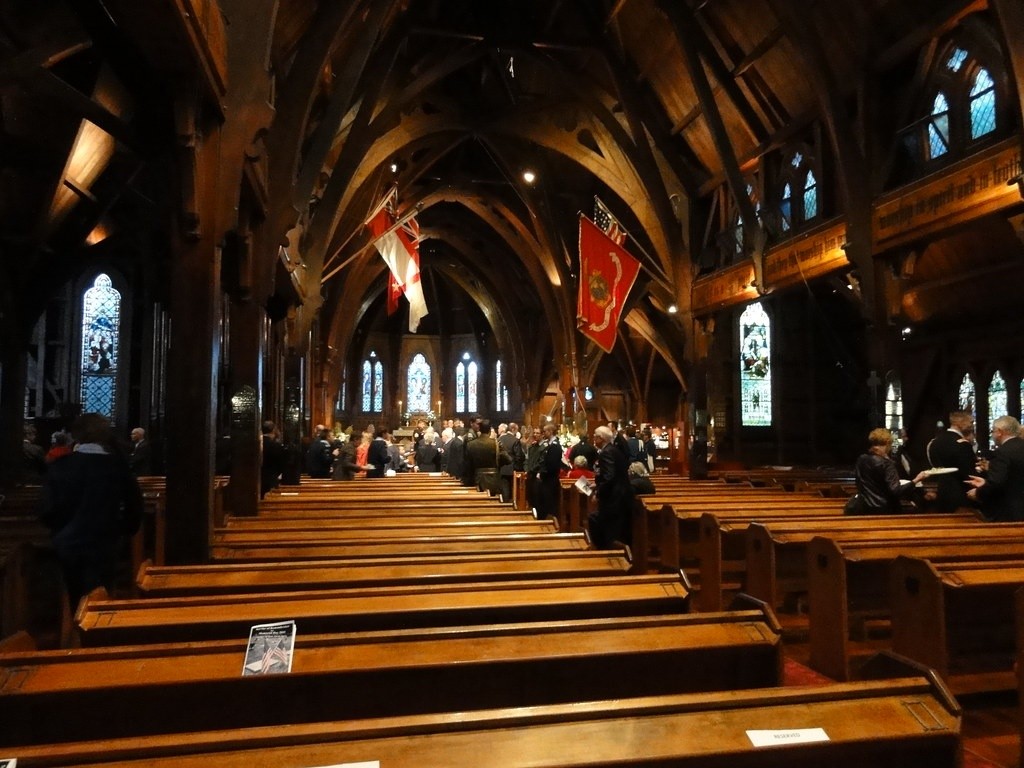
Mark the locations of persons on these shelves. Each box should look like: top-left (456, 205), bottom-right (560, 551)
top-left (261, 420), bottom-right (416, 499)
top-left (130, 427), bottom-right (146, 450)
top-left (413, 415), bottom-right (657, 550)
top-left (843, 412), bottom-right (1024, 522)
top-left (45, 433), bottom-right (71, 458)
top-left (71, 413), bottom-right (110, 455)
top-left (22, 424), bottom-right (43, 461)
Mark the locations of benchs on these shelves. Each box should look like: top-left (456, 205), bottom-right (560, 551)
top-left (0, 470), bottom-right (1024, 768)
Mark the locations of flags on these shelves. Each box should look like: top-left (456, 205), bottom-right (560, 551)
top-left (373, 212), bottom-right (429, 333)
top-left (575, 213), bottom-right (642, 355)
top-left (593, 199), bottom-right (628, 247)
top-left (363, 187), bottom-right (402, 316)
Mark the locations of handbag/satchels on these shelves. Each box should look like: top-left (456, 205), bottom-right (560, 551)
top-left (845, 476), bottom-right (891, 515)
